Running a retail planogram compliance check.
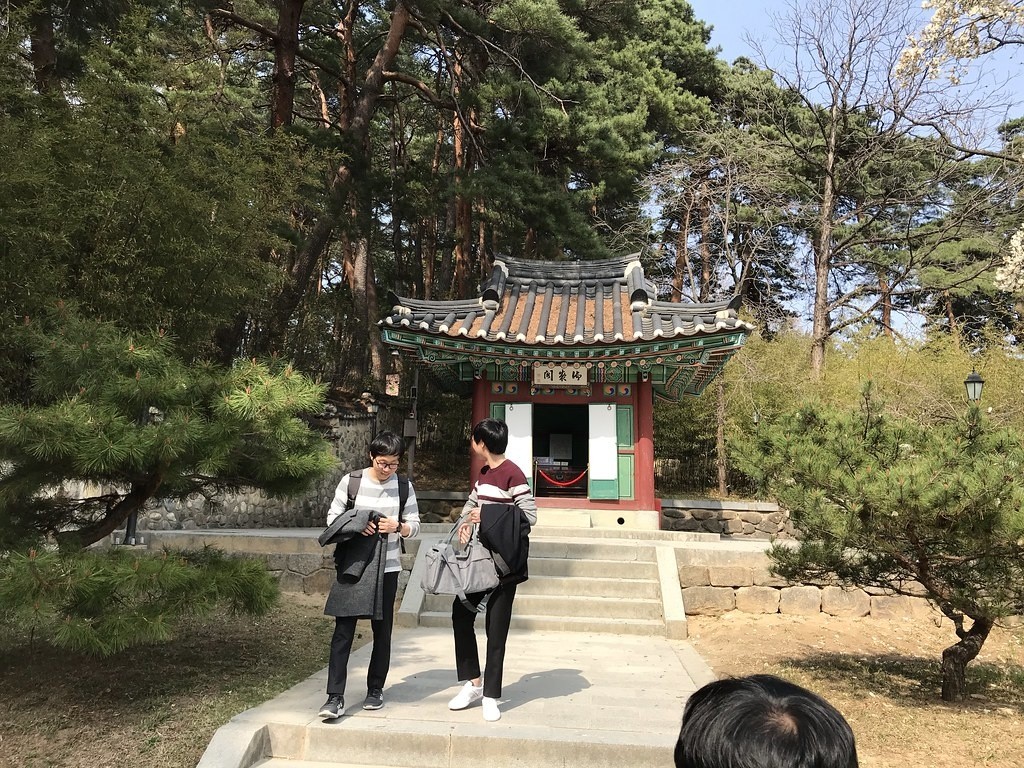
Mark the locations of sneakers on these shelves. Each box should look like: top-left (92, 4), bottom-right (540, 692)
top-left (362, 687), bottom-right (384, 710)
top-left (318, 694), bottom-right (345, 718)
top-left (481, 695), bottom-right (501, 722)
top-left (448, 677), bottom-right (484, 710)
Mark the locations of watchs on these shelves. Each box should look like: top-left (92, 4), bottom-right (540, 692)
top-left (396, 521), bottom-right (402, 532)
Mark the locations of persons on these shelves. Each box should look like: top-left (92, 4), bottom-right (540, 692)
top-left (318, 430), bottom-right (421, 719)
top-left (448, 417), bottom-right (538, 721)
top-left (674, 673), bottom-right (859, 768)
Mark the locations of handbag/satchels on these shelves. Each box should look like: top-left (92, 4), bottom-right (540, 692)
top-left (419, 514), bottom-right (500, 612)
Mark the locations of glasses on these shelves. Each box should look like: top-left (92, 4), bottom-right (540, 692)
top-left (373, 458), bottom-right (401, 469)
